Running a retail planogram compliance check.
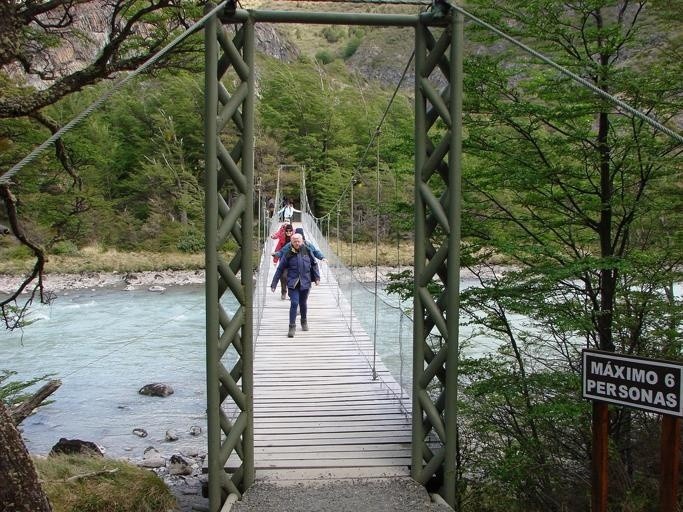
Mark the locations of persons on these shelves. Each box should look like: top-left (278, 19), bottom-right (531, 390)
top-left (272, 224), bottom-right (296, 300)
top-left (276, 201), bottom-right (303, 226)
top-left (271, 228), bottom-right (327, 266)
top-left (270, 217), bottom-right (291, 240)
top-left (269, 233), bottom-right (320, 337)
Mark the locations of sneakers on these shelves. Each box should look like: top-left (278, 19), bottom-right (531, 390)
top-left (288, 324), bottom-right (295, 337)
top-left (301, 319), bottom-right (308, 331)
top-left (281, 291), bottom-right (286, 299)
